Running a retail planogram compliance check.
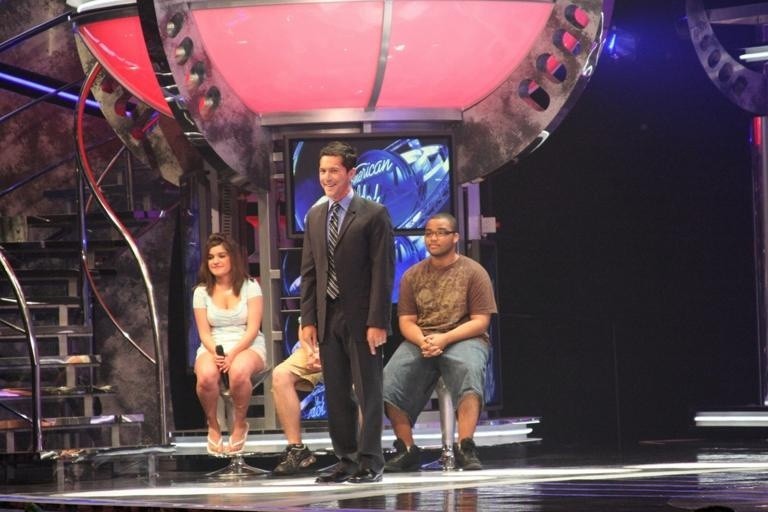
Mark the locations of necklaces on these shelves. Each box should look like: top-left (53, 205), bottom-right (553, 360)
top-left (219, 286), bottom-right (235, 296)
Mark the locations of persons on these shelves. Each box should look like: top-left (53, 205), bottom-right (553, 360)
top-left (382, 213), bottom-right (499, 471)
top-left (190, 231), bottom-right (267, 458)
top-left (269, 313), bottom-right (363, 475)
top-left (299, 141), bottom-right (398, 484)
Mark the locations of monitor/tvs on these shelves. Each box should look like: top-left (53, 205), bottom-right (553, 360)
top-left (280, 309), bottom-right (303, 359)
top-left (388, 233), bottom-right (462, 313)
top-left (284, 129), bottom-right (461, 240)
top-left (279, 246), bottom-right (306, 300)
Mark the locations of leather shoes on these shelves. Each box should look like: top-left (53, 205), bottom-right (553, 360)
top-left (349, 464), bottom-right (385, 483)
top-left (317, 460), bottom-right (359, 484)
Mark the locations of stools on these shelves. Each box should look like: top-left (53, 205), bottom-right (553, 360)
top-left (420, 378), bottom-right (464, 471)
top-left (204, 369), bottom-right (270, 478)
top-left (315, 383), bottom-right (364, 474)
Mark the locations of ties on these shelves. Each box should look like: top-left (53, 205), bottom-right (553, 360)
top-left (325, 202), bottom-right (341, 300)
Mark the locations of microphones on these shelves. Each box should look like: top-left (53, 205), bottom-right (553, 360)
top-left (216, 345), bottom-right (231, 388)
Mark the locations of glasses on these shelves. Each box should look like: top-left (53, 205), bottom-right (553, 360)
top-left (425, 230), bottom-right (456, 237)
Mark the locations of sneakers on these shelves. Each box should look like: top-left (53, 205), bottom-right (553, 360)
top-left (273, 446), bottom-right (314, 475)
top-left (383, 439), bottom-right (423, 472)
top-left (454, 437), bottom-right (482, 470)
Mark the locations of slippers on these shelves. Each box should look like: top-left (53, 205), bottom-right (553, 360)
top-left (226, 423), bottom-right (249, 455)
top-left (206, 421), bottom-right (223, 456)
